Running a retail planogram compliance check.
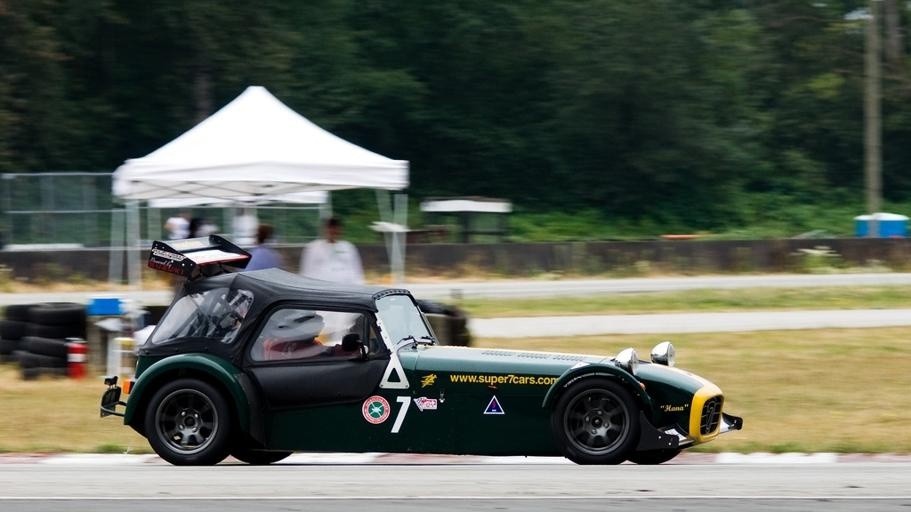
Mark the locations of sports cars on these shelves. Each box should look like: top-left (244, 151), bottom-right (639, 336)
top-left (100, 233), bottom-right (744, 467)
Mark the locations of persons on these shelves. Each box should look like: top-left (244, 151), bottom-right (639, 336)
top-left (299, 216), bottom-right (364, 285)
top-left (241, 226), bottom-right (283, 273)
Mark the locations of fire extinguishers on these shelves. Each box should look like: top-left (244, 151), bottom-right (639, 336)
top-left (64, 337), bottom-right (90, 380)
